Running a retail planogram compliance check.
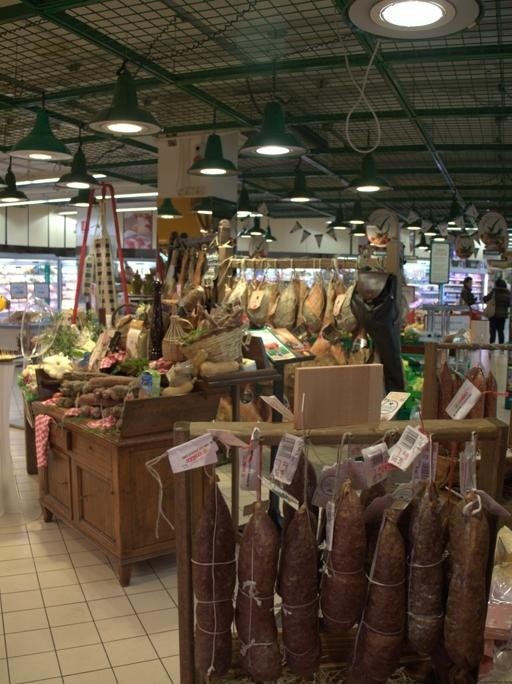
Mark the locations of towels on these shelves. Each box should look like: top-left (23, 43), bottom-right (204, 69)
top-left (33, 415), bottom-right (55, 468)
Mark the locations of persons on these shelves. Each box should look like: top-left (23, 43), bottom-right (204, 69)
top-left (461, 277), bottom-right (474, 305)
top-left (483, 279), bottom-right (511, 344)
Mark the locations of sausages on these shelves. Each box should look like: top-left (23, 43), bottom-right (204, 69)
top-left (438, 362), bottom-right (497, 419)
top-left (190, 450), bottom-right (491, 684)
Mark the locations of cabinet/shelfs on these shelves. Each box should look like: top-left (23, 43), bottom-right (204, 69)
top-left (29, 402), bottom-right (174, 587)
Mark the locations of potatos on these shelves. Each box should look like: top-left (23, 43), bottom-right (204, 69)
top-left (55, 370), bottom-right (136, 429)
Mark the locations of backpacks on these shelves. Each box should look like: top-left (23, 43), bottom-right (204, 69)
top-left (347, 268), bottom-right (398, 333)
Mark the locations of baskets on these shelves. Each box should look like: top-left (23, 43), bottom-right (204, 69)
top-left (178, 310), bottom-right (245, 366)
top-left (160, 314), bottom-right (185, 364)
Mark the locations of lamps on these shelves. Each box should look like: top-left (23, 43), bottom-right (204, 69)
top-left (9, 90), bottom-right (73, 161)
top-left (55, 125), bottom-right (99, 189)
top-left (238, 160), bottom-right (479, 250)
top-left (0, 155), bottom-right (29, 203)
top-left (344, 0), bottom-right (485, 43)
top-left (187, 106), bottom-right (238, 177)
top-left (157, 198), bottom-right (213, 219)
top-left (238, 61), bottom-right (308, 160)
top-left (69, 189), bottom-right (99, 207)
top-left (89, 54), bottom-right (162, 136)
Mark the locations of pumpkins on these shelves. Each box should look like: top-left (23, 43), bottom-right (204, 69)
top-left (200, 360), bottom-right (239, 381)
top-left (190, 348), bottom-right (209, 376)
top-left (162, 376), bottom-right (198, 398)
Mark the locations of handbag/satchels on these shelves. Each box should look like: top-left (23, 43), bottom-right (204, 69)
top-left (483, 288), bottom-right (496, 319)
